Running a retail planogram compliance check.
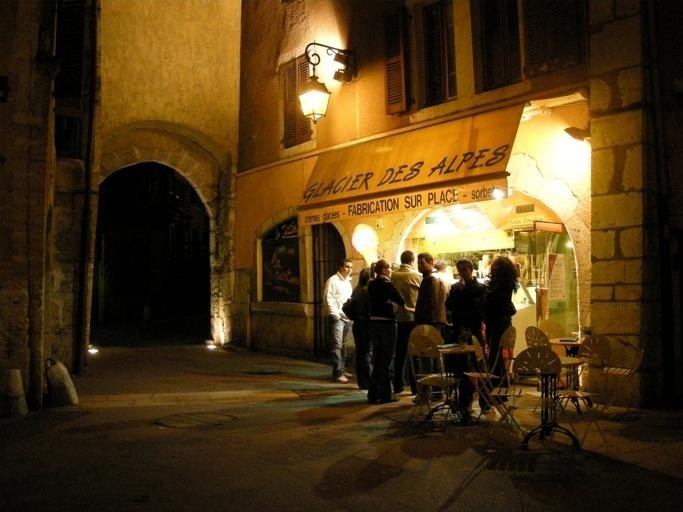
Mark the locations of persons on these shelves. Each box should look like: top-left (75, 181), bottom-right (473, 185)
top-left (321, 251), bottom-right (519, 425)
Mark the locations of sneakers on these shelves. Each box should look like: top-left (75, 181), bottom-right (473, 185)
top-left (335, 372), bottom-right (352, 383)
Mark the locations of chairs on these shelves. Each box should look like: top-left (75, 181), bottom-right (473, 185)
top-left (404, 321), bottom-right (650, 453)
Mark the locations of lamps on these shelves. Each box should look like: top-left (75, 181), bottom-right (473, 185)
top-left (549, 118), bottom-right (593, 163)
top-left (295, 40), bottom-right (357, 123)
